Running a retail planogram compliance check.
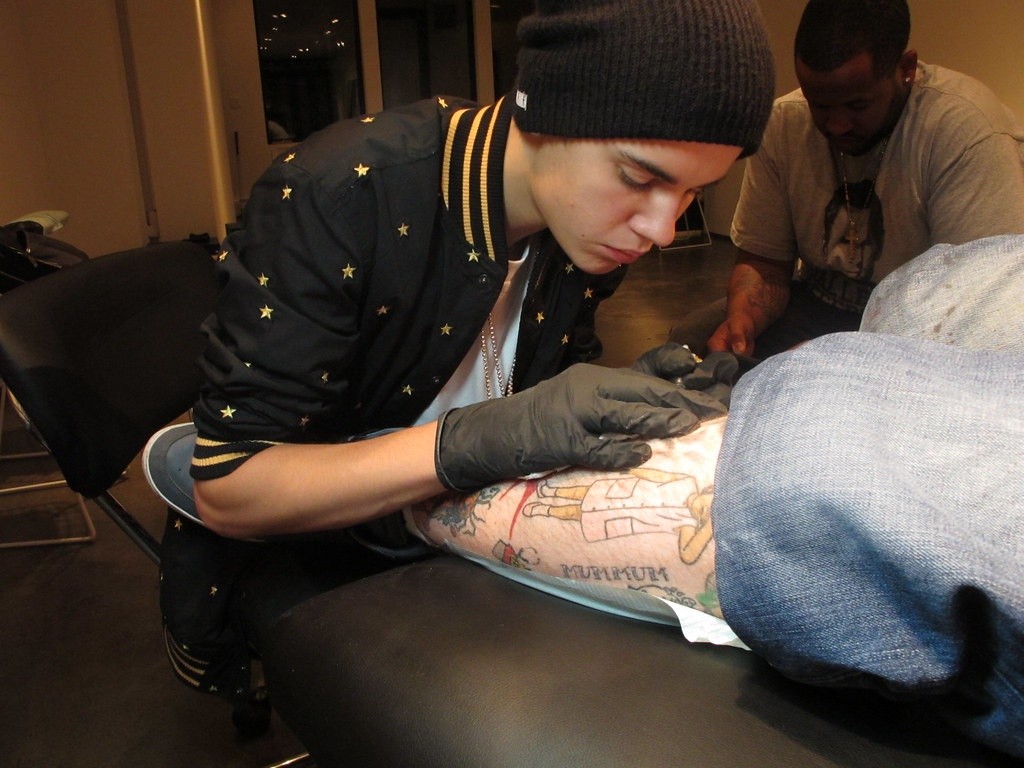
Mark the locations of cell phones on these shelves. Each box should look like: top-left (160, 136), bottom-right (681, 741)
top-left (735, 353), bottom-right (761, 367)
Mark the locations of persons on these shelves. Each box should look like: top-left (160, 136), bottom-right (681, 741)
top-left (822, 182), bottom-right (886, 320)
top-left (156, 0), bottom-right (780, 746)
top-left (140, 232), bottom-right (1023, 756)
top-left (706, 0), bottom-right (1024, 360)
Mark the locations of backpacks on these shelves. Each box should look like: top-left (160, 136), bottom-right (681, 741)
top-left (1, 226), bottom-right (89, 293)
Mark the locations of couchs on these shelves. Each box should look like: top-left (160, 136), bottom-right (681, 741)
top-left (269, 553), bottom-right (1024, 768)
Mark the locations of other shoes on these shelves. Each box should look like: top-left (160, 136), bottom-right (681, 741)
top-left (143, 420), bottom-right (438, 558)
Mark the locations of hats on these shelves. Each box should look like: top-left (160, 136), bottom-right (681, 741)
top-left (512, 0), bottom-right (776, 160)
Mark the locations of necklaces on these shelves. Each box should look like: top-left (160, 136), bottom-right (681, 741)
top-left (479, 230), bottom-right (544, 401)
top-left (837, 131), bottom-right (894, 258)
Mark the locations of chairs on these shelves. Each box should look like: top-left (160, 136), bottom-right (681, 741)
top-left (0, 238), bottom-right (219, 568)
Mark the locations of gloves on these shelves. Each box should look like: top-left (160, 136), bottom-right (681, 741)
top-left (434, 364), bottom-right (728, 492)
top-left (627, 343), bottom-right (737, 410)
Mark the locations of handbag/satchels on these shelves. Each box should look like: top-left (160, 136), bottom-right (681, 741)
top-left (189, 233), bottom-right (210, 243)
